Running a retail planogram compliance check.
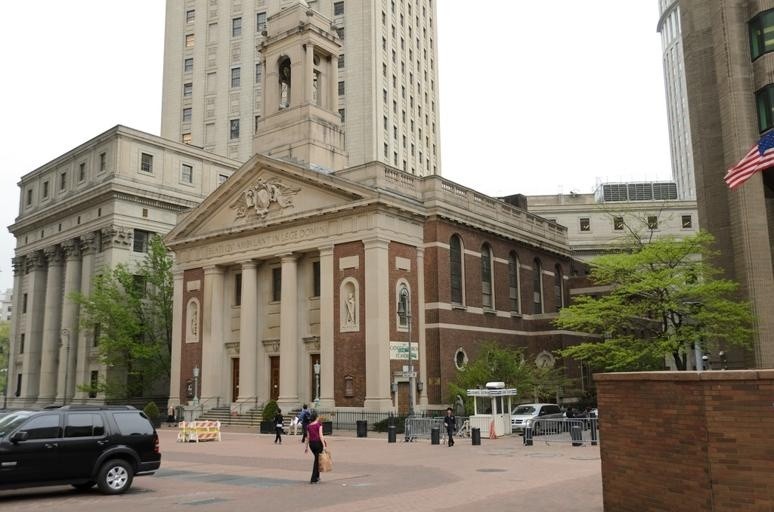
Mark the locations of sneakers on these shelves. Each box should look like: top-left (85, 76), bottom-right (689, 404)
top-left (311, 478), bottom-right (321, 484)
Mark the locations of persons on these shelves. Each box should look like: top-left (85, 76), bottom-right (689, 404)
top-left (297, 405), bottom-right (310, 443)
top-left (273, 408), bottom-right (282, 445)
top-left (303, 413), bottom-right (325, 484)
top-left (443, 407), bottom-right (456, 446)
top-left (566, 408), bottom-right (584, 418)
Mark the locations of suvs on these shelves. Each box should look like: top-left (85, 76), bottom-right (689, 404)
top-left (0, 399), bottom-right (161, 495)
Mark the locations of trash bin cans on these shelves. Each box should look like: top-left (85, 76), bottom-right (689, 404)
top-left (356, 420), bottom-right (367, 437)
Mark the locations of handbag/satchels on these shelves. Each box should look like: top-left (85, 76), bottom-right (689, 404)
top-left (317, 450), bottom-right (334, 473)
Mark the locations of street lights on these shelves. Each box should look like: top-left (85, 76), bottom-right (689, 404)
top-left (58, 327), bottom-right (71, 406)
top-left (396, 288), bottom-right (417, 418)
top-left (313, 359), bottom-right (321, 407)
top-left (191, 364), bottom-right (200, 405)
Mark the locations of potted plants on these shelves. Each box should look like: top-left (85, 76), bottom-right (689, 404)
top-left (259, 397), bottom-right (279, 435)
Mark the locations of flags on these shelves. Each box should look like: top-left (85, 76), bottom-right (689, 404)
top-left (722, 130), bottom-right (774, 190)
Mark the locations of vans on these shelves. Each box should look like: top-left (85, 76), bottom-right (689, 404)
top-left (511, 403), bottom-right (563, 434)
